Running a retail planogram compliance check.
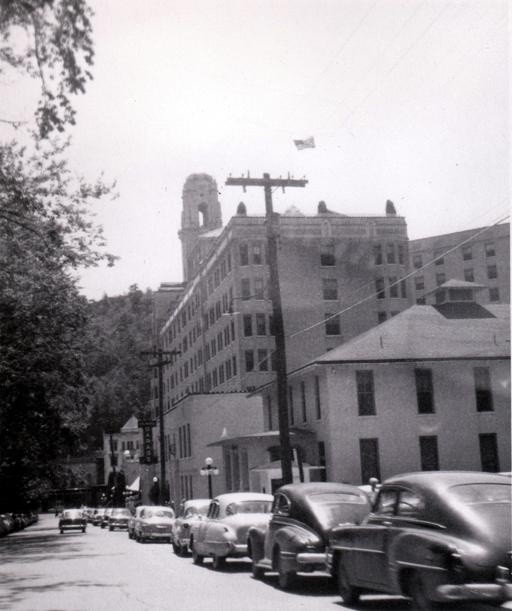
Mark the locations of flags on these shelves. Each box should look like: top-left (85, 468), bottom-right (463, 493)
top-left (292, 136), bottom-right (316, 151)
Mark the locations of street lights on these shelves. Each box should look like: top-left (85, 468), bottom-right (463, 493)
top-left (153, 477), bottom-right (158, 506)
top-left (200, 456), bottom-right (219, 498)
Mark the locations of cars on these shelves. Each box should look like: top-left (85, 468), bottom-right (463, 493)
top-left (246, 470), bottom-right (512, 611)
top-left (0, 511), bottom-right (39, 537)
top-left (128, 491), bottom-right (276, 570)
top-left (59, 507), bottom-right (133, 534)
